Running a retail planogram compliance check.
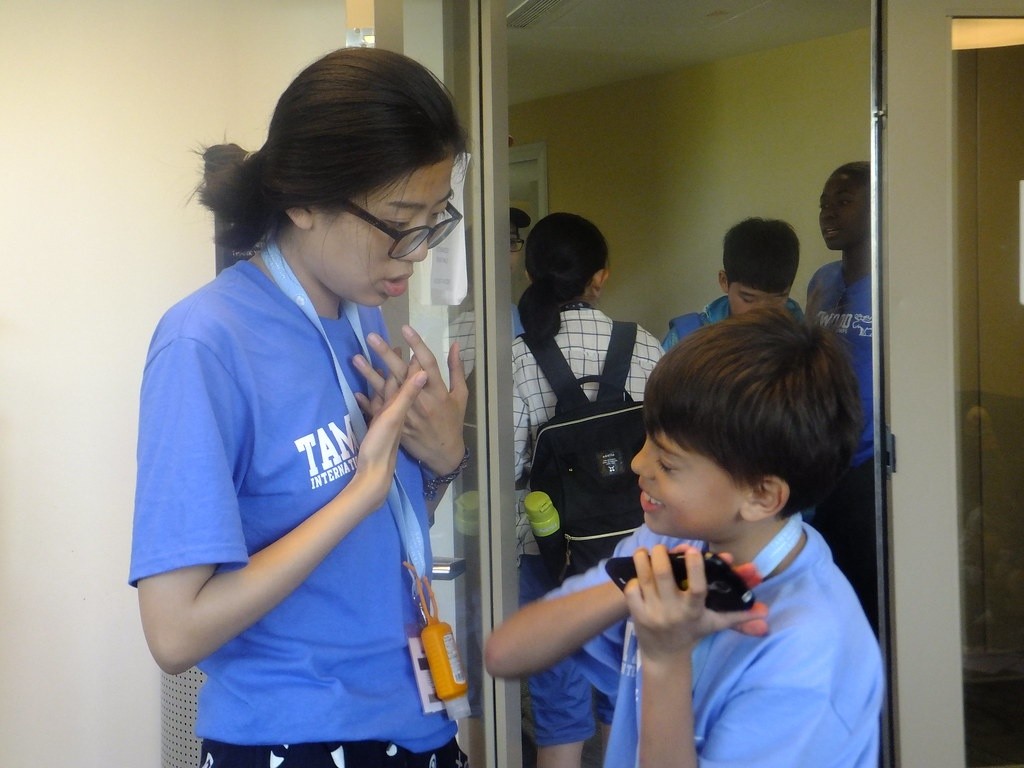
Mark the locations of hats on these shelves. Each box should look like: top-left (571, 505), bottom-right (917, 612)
top-left (509, 206), bottom-right (531, 228)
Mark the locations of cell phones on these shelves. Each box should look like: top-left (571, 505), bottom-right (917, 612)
top-left (604, 551), bottom-right (757, 615)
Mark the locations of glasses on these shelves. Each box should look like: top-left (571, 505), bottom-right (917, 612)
top-left (334, 194), bottom-right (464, 259)
top-left (509, 237), bottom-right (525, 252)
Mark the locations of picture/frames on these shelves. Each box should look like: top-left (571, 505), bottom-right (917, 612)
top-left (510, 142), bottom-right (550, 221)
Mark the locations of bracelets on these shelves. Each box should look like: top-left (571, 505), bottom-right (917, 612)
top-left (419, 445), bottom-right (471, 502)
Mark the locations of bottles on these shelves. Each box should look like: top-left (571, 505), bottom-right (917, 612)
top-left (524, 490), bottom-right (560, 537)
top-left (454, 490), bottom-right (481, 536)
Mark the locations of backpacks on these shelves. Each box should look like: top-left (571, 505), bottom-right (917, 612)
top-left (516, 320), bottom-right (646, 591)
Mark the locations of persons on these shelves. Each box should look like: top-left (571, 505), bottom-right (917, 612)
top-left (444, 227), bottom-right (485, 768)
top-left (129, 48), bottom-right (470, 768)
top-left (485, 302), bottom-right (885, 768)
top-left (508, 160), bottom-right (879, 768)
